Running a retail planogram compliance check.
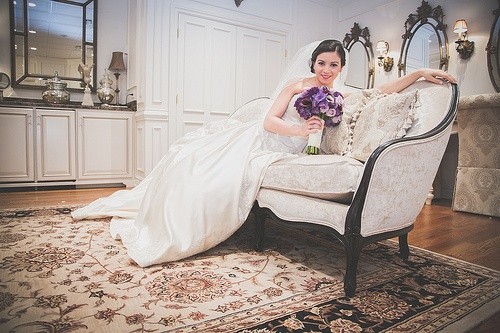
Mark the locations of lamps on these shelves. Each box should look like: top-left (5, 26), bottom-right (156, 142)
top-left (376, 40), bottom-right (394, 72)
top-left (106, 50), bottom-right (126, 106)
top-left (453, 19), bottom-right (475, 60)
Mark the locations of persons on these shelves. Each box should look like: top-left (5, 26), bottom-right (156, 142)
top-left (71, 40), bottom-right (459, 268)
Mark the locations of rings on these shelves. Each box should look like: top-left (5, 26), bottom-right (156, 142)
top-left (309, 125), bottom-right (313, 129)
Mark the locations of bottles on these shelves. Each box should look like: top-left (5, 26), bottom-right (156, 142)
top-left (97, 70), bottom-right (116, 106)
top-left (42, 70), bottom-right (70, 108)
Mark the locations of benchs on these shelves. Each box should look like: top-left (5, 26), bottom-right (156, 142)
top-left (227, 74), bottom-right (461, 301)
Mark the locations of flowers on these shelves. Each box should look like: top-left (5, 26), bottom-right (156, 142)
top-left (292, 85), bottom-right (344, 156)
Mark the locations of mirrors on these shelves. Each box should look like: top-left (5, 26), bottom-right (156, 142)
top-left (9, 0), bottom-right (99, 94)
top-left (397, 0), bottom-right (450, 80)
top-left (485, 7), bottom-right (500, 93)
top-left (343, 22), bottom-right (375, 91)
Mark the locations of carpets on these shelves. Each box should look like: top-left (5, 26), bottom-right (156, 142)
top-left (0, 204), bottom-right (500, 333)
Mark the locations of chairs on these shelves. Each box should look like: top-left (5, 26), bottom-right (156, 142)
top-left (450, 93), bottom-right (500, 220)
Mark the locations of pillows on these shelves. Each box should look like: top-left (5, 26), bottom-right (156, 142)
top-left (348, 89), bottom-right (423, 163)
top-left (319, 89), bottom-right (381, 156)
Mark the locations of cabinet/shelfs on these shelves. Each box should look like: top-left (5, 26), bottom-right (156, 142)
top-left (0, 106), bottom-right (134, 194)
